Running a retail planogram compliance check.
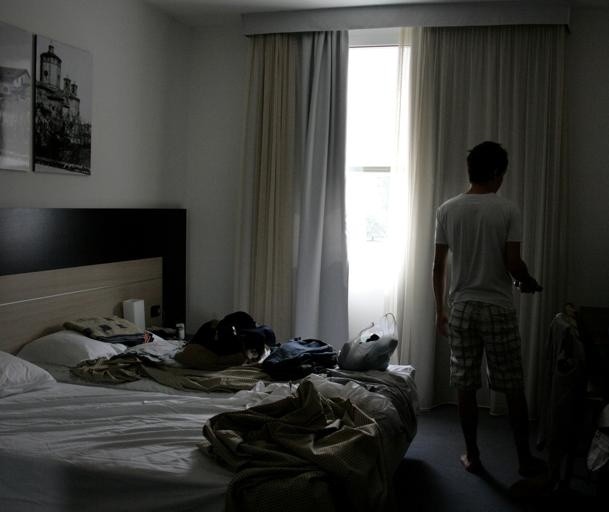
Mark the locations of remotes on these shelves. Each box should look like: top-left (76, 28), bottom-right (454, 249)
top-left (514, 281), bottom-right (543, 292)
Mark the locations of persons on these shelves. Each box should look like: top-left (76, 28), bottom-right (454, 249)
top-left (431, 139), bottom-right (544, 477)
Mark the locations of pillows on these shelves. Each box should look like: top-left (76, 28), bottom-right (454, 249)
top-left (0, 349), bottom-right (57, 398)
top-left (15, 328), bottom-right (128, 369)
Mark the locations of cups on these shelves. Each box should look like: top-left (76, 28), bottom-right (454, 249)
top-left (176, 323), bottom-right (185, 341)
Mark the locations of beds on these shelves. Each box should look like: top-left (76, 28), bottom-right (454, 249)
top-left (0, 257), bottom-right (417, 512)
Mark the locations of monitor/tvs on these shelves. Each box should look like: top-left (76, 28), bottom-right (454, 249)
top-left (579, 306), bottom-right (609, 346)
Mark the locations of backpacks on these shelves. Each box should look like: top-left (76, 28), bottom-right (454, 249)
top-left (263, 337), bottom-right (337, 377)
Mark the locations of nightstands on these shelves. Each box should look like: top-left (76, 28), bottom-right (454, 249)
top-left (151, 325), bottom-right (193, 343)
